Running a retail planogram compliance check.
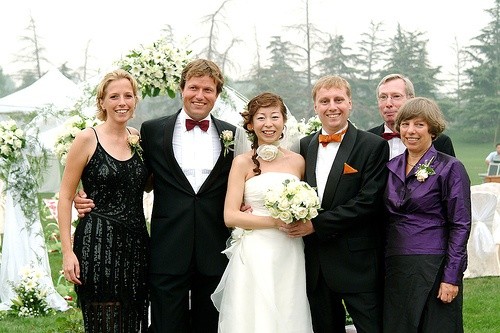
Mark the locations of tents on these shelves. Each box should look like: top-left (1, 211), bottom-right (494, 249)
top-left (0, 66), bottom-right (86, 113)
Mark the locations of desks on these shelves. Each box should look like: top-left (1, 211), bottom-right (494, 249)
top-left (470, 182), bottom-right (500, 244)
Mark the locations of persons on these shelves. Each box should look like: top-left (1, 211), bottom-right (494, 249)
top-left (240, 76), bottom-right (390, 333)
top-left (485, 144), bottom-right (500, 165)
top-left (57, 69), bottom-right (153, 333)
top-left (366, 74), bottom-right (456, 163)
top-left (74, 59), bottom-right (240, 333)
top-left (382, 97), bottom-right (472, 333)
top-left (223, 93), bottom-right (314, 333)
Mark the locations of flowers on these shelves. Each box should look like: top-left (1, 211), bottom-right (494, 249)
top-left (414, 156), bottom-right (436, 182)
top-left (231, 226), bottom-right (244, 239)
top-left (264, 176), bottom-right (324, 224)
top-left (6, 266), bottom-right (56, 318)
top-left (299, 114), bottom-right (322, 135)
top-left (53, 39), bottom-right (238, 167)
top-left (0, 119), bottom-right (27, 162)
top-left (256, 144), bottom-right (279, 162)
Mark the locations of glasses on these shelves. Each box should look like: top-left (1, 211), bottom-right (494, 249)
top-left (378, 93), bottom-right (413, 101)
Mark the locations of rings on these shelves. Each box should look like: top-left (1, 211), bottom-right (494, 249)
top-left (453, 297), bottom-right (456, 298)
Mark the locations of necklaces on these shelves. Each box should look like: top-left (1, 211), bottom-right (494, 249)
top-left (407, 162), bottom-right (415, 166)
top-left (255, 143), bottom-right (281, 161)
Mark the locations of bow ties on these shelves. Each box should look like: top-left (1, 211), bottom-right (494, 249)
top-left (186, 119), bottom-right (209, 132)
top-left (382, 132), bottom-right (401, 140)
top-left (319, 129), bottom-right (347, 147)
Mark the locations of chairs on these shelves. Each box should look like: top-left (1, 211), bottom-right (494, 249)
top-left (463, 192), bottom-right (500, 279)
